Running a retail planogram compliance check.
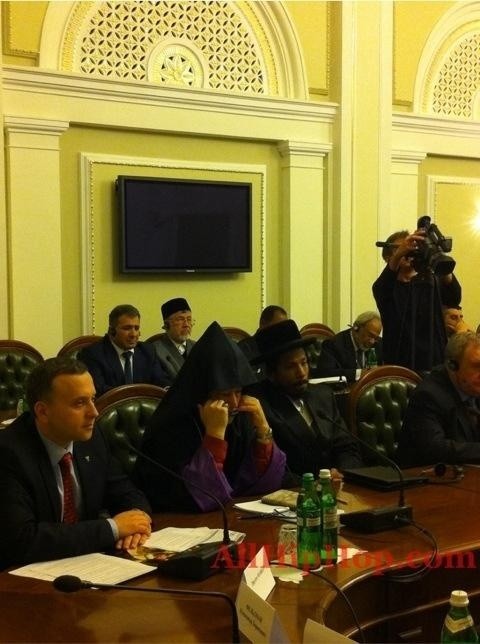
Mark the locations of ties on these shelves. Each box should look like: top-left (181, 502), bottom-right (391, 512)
top-left (122, 351), bottom-right (134, 385)
top-left (59, 452), bottom-right (78, 524)
top-left (357, 349), bottom-right (363, 368)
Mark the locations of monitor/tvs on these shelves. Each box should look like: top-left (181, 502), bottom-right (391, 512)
top-left (118, 175), bottom-right (251, 274)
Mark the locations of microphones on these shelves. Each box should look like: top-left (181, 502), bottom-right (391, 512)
top-left (115, 436), bottom-right (238, 578)
top-left (53, 576), bottom-right (240, 644)
top-left (315, 408), bottom-right (412, 532)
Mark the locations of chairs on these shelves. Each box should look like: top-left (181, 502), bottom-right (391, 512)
top-left (0, 338), bottom-right (45, 423)
top-left (93, 383), bottom-right (168, 470)
top-left (295, 323), bottom-right (336, 387)
top-left (56, 335), bottom-right (105, 361)
top-left (220, 326), bottom-right (252, 344)
top-left (349, 365), bottom-right (423, 466)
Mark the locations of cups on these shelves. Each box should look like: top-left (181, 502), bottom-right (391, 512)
top-left (277, 523), bottom-right (298, 569)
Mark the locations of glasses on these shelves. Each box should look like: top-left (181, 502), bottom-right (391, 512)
top-left (168, 316), bottom-right (197, 326)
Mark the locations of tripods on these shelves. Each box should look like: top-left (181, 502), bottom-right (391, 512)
top-left (393, 271), bottom-right (450, 372)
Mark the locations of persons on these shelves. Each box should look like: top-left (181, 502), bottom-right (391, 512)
top-left (373, 230), bottom-right (462, 373)
top-left (1, 357), bottom-right (152, 568)
top-left (78, 298), bottom-right (479, 514)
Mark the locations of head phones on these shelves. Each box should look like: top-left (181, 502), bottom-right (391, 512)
top-left (448, 359), bottom-right (459, 371)
top-left (162, 321), bottom-right (170, 330)
top-left (418, 463), bottom-right (464, 484)
top-left (108, 327), bottom-right (116, 335)
top-left (353, 320), bottom-right (360, 331)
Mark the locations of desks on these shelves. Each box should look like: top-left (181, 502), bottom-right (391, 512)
top-left (1, 463), bottom-right (480, 644)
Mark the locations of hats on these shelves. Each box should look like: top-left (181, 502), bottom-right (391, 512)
top-left (246, 320), bottom-right (316, 365)
top-left (161, 298), bottom-right (192, 321)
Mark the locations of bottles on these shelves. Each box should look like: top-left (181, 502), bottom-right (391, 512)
top-left (297, 469), bottom-right (337, 570)
top-left (440, 585), bottom-right (480, 643)
top-left (365, 347), bottom-right (377, 371)
top-left (17, 398), bottom-right (24, 419)
